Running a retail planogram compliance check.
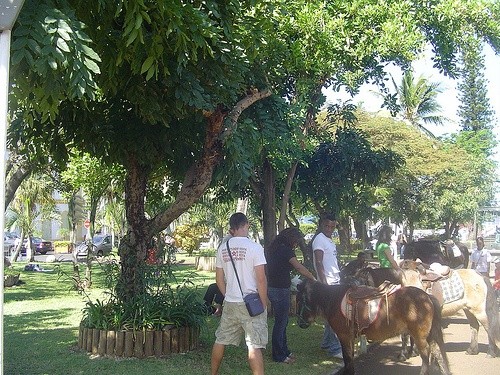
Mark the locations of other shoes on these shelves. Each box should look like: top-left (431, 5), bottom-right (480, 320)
top-left (289, 353), bottom-right (296, 359)
top-left (284, 357), bottom-right (296, 363)
top-left (335, 352), bottom-right (344, 359)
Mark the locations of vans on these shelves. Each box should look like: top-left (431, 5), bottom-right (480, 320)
top-left (76, 235), bottom-right (119, 256)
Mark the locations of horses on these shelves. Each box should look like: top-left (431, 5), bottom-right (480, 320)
top-left (403, 237), bottom-right (469, 269)
top-left (294, 276), bottom-right (450, 375)
top-left (339, 251), bottom-right (500, 363)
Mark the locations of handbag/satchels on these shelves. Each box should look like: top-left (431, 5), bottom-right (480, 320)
top-left (244, 293), bottom-right (264, 317)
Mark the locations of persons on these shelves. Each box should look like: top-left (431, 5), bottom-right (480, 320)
top-left (492, 258), bottom-right (500, 299)
top-left (165, 234), bottom-right (175, 254)
top-left (496, 225), bottom-right (500, 243)
top-left (210, 213), bottom-right (269, 375)
top-left (202, 213), bottom-right (368, 362)
top-left (374, 226), bottom-right (408, 270)
top-left (471, 237), bottom-right (491, 277)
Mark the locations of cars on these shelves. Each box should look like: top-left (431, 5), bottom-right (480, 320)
top-left (3, 230), bottom-right (54, 256)
